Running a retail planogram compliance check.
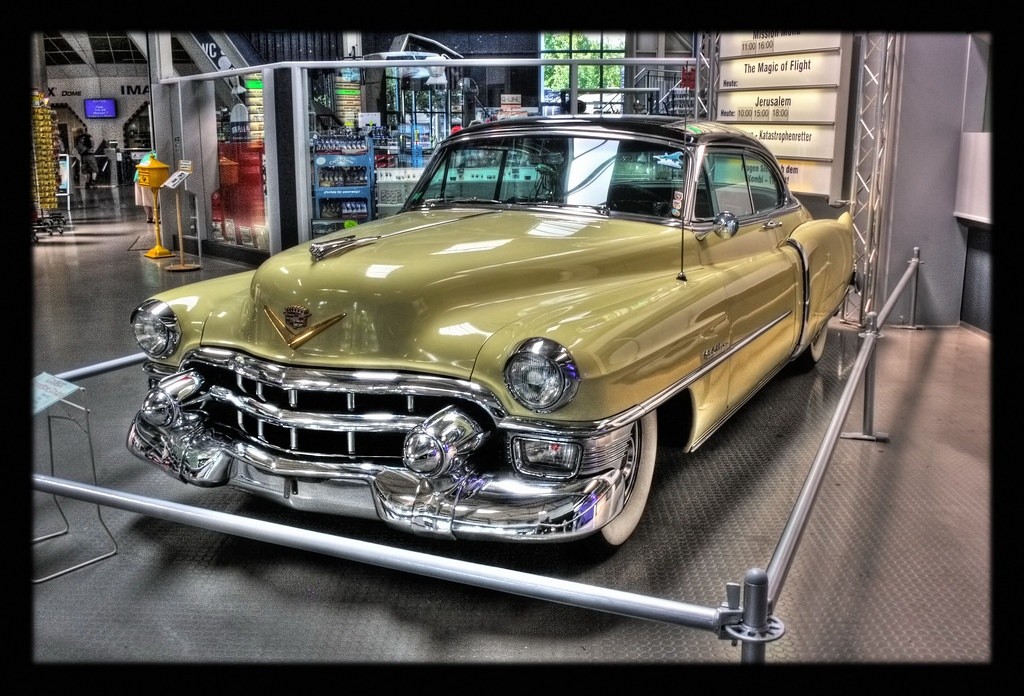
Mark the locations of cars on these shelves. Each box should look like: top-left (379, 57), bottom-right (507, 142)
top-left (127, 116), bottom-right (855, 560)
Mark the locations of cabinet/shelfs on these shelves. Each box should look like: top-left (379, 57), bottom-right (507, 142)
top-left (313, 136), bottom-right (399, 222)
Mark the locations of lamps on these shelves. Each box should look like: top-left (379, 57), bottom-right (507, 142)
top-left (227, 67), bottom-right (247, 94)
top-left (425, 57), bottom-right (447, 84)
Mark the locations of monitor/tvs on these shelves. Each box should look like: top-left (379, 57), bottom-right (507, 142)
top-left (84, 98), bottom-right (119, 120)
